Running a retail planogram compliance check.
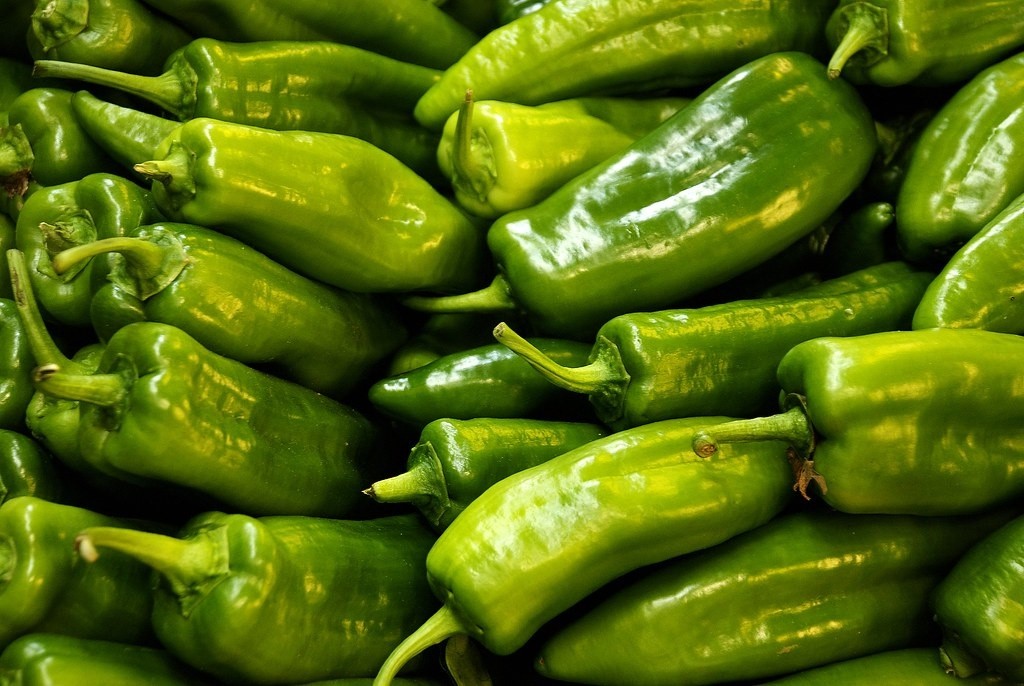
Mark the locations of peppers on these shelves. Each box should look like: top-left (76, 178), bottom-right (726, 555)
top-left (0, 0), bottom-right (1024, 686)
